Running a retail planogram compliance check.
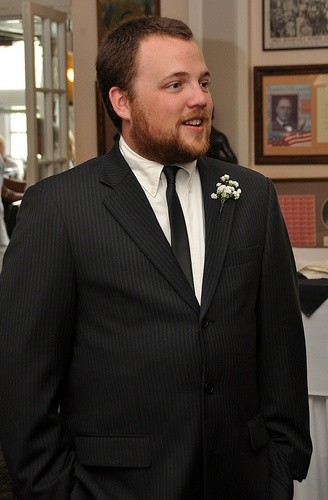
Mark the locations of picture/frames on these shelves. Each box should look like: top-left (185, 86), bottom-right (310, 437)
top-left (262, 0), bottom-right (328, 51)
top-left (254, 64), bottom-right (328, 165)
top-left (97, 0), bottom-right (161, 49)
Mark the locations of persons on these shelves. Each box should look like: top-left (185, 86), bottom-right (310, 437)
top-left (0, 135), bottom-right (19, 180)
top-left (0, 153), bottom-right (10, 253)
top-left (204, 106), bottom-right (238, 165)
top-left (272, 98), bottom-right (297, 132)
top-left (286, 10), bottom-right (313, 38)
top-left (0, 16), bottom-right (313, 500)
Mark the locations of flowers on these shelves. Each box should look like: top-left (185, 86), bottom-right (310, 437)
top-left (211, 174), bottom-right (242, 217)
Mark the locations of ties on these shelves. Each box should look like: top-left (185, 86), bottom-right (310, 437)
top-left (162, 166), bottom-right (194, 293)
